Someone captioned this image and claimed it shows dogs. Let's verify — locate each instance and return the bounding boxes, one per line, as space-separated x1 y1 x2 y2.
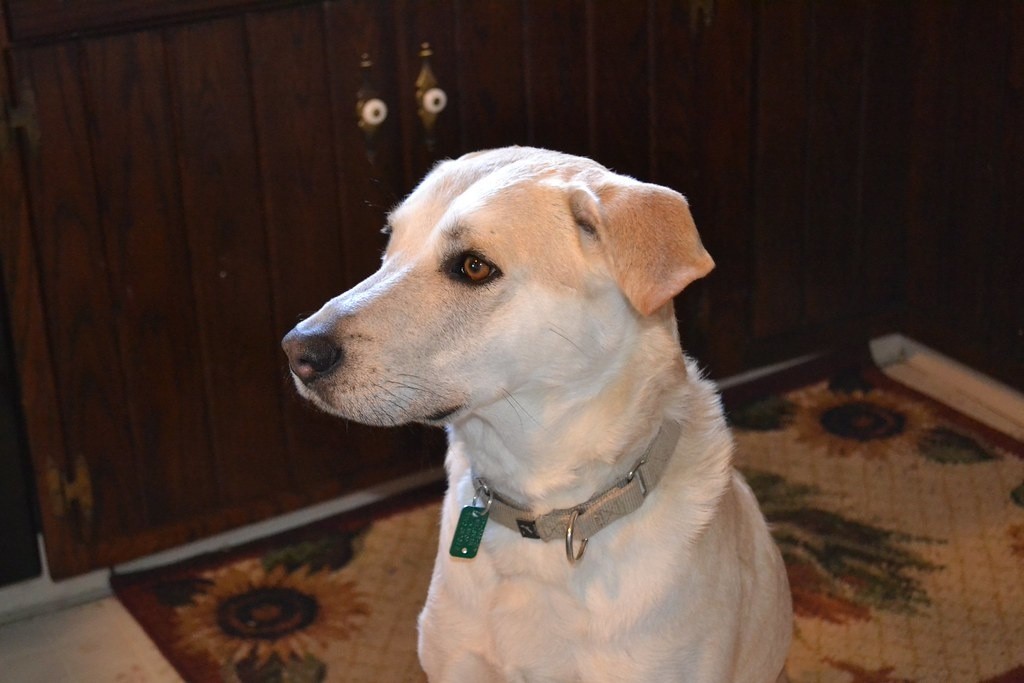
279 143 795 683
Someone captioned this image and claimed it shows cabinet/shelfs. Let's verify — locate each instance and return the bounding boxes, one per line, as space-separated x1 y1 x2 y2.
0 0 1024 567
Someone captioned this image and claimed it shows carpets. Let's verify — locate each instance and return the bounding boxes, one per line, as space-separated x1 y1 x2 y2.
110 330 1024 683
